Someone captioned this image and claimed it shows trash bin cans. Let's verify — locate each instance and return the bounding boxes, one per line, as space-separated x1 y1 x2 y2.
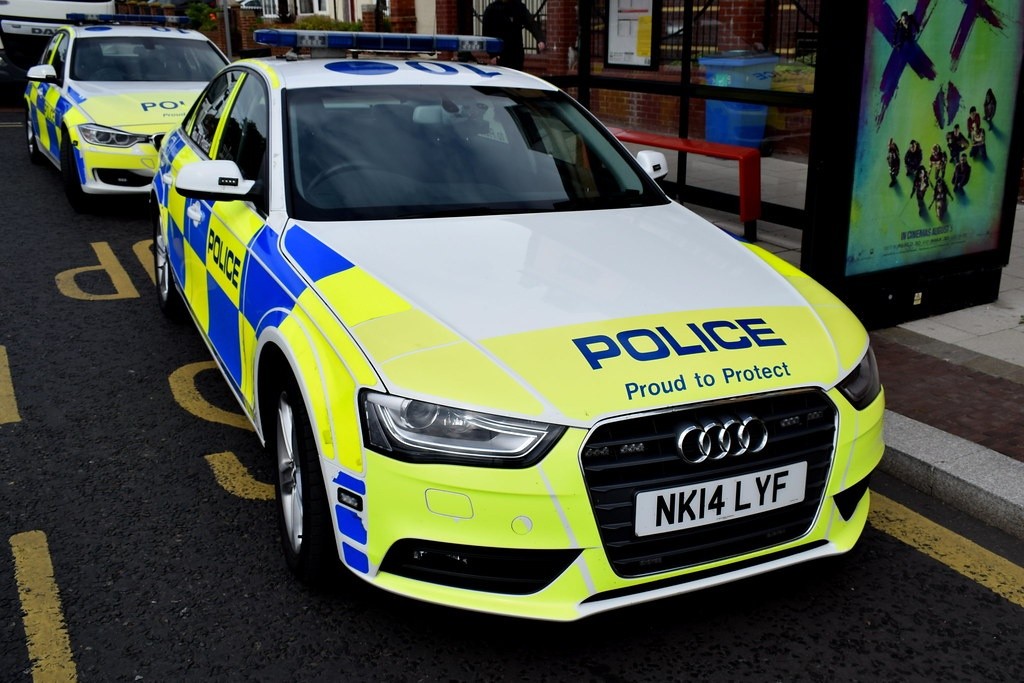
698 51 779 148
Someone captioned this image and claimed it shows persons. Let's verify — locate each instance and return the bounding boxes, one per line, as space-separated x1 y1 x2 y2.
910 165 935 222
970 120 988 162
897 10 911 29
905 140 922 176
968 106 980 138
139 42 192 81
297 103 439 195
983 89 996 130
77 42 105 78
928 144 947 179
887 139 901 187
929 178 953 220
946 124 969 165
483 0 546 71
951 153 971 197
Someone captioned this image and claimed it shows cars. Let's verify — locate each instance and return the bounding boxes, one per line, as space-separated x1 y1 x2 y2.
149 27 888 625
0 0 120 72
22 10 238 206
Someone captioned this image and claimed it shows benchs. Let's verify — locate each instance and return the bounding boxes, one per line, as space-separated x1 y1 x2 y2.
583 127 762 244
